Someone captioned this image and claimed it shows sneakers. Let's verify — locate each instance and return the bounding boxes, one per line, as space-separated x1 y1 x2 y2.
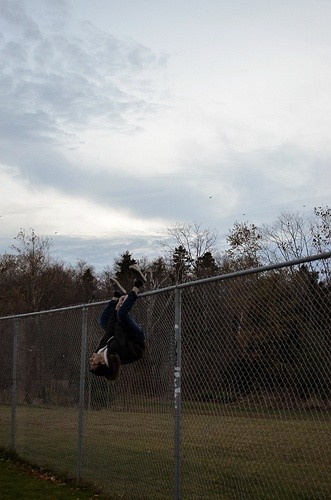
129 266 146 283
109 277 128 296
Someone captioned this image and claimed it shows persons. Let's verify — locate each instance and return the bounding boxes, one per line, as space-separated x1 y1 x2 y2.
85 263 149 381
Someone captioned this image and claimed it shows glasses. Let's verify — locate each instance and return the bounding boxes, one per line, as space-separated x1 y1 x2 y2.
89 362 99 366
